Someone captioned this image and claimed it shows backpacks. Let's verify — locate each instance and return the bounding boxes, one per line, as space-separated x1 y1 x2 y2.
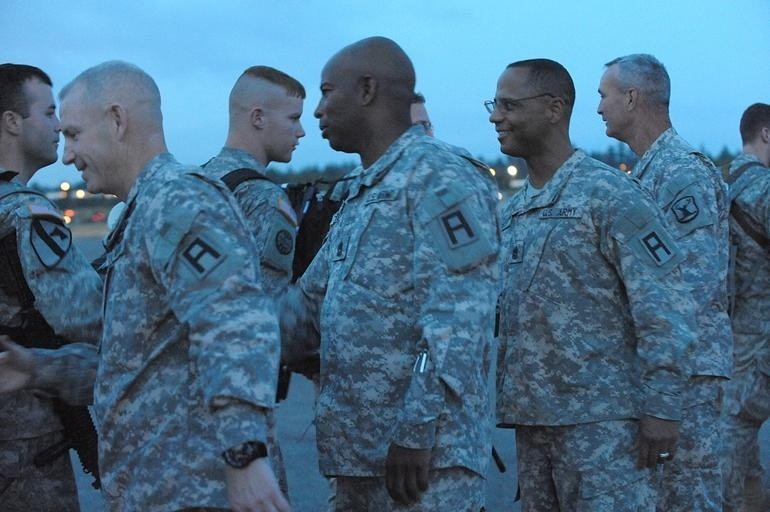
278 175 358 374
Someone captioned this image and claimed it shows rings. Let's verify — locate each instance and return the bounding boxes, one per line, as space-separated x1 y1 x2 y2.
659 449 671 458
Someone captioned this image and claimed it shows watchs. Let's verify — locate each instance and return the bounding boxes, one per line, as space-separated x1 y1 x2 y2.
223 440 266 470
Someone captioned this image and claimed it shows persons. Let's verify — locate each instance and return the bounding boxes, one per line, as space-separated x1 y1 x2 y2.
470 58 689 509
197 63 304 302
594 57 734 511
278 33 495 512
406 89 437 144
2 61 121 510
723 102 769 510
3 58 281 508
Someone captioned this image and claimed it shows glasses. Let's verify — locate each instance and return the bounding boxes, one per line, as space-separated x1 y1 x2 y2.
485 93 555 114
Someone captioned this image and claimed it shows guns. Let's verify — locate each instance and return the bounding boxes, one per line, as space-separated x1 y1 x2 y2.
3 228 102 489
